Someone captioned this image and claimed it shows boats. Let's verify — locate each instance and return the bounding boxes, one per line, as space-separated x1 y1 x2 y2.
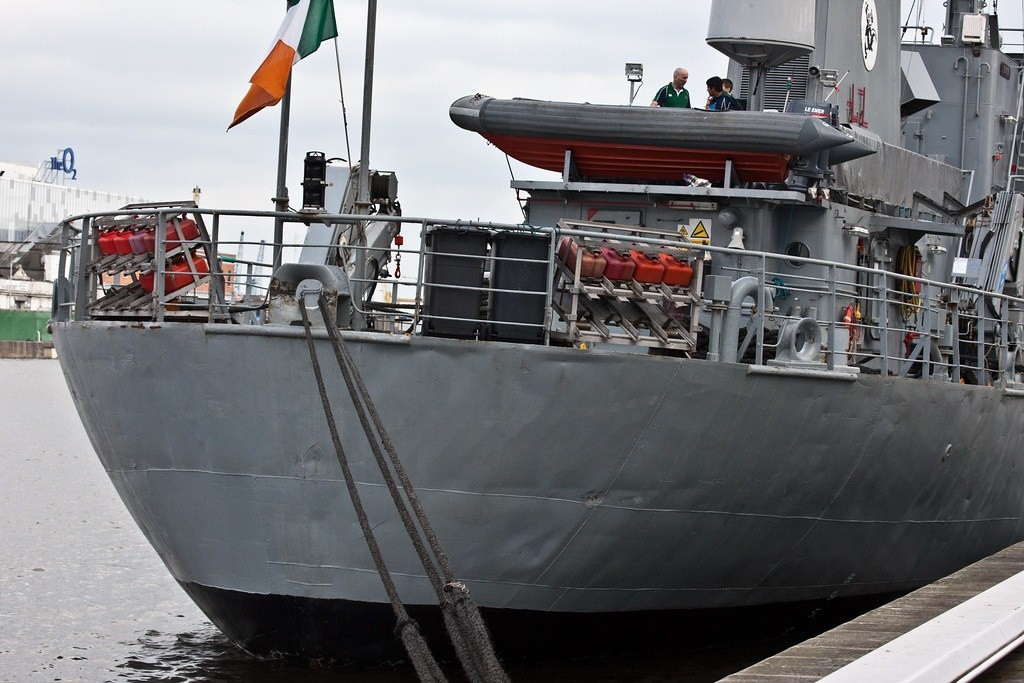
48 0 1024 674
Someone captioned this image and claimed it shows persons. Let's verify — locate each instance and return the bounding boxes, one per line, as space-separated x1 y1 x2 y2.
650 68 691 108
706 76 744 111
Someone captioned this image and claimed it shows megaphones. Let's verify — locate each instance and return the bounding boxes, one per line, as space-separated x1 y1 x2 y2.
808 66 820 77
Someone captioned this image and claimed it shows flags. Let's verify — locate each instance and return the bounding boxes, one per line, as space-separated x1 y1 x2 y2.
226 0 339 133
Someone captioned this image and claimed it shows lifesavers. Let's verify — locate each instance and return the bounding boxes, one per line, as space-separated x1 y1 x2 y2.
841 304 860 349
